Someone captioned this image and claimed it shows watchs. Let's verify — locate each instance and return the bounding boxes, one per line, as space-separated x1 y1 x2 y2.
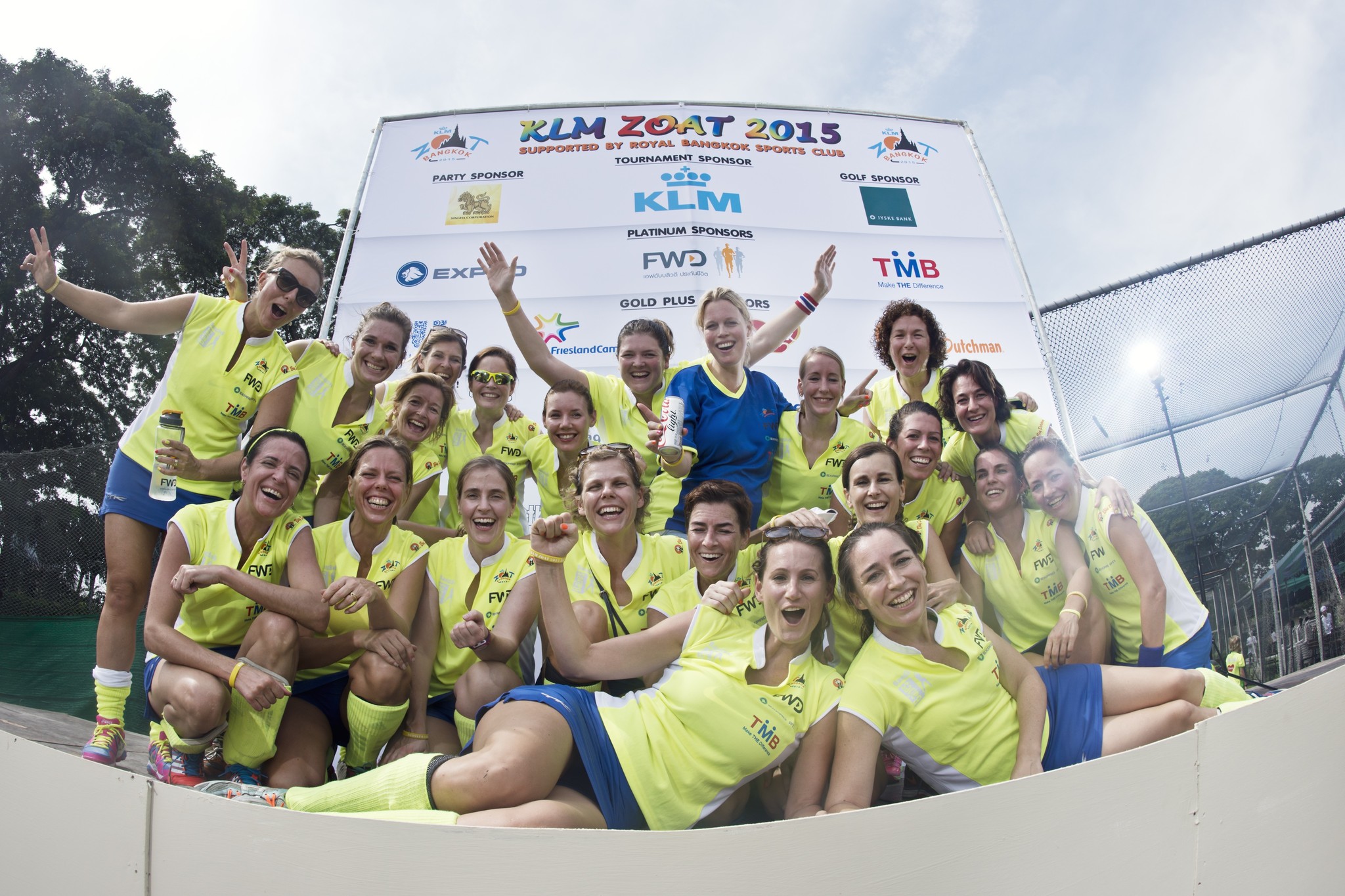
469 627 491 651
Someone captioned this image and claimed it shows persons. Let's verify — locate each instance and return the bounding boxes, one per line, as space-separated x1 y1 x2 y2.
861 299 1038 453
756 346 960 538
317 338 467 548
371 325 524 468
476 241 836 534
221 239 412 526
825 522 1286 817
142 427 332 786
383 456 542 764
524 380 648 532
960 444 1111 668
1226 605 1334 689
647 480 828 829
935 359 1135 556
437 346 543 537
749 442 972 677
1022 437 1212 669
20 226 324 784
193 510 840 829
827 401 970 565
266 436 430 788
635 286 878 541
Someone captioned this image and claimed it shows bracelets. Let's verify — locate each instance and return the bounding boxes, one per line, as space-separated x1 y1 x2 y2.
1139 644 1165 667
45 275 60 294
795 292 818 315
770 515 783 528
966 520 985 527
228 662 246 689
403 730 428 740
1065 591 1088 615
502 300 520 316
530 548 565 563
1059 609 1081 620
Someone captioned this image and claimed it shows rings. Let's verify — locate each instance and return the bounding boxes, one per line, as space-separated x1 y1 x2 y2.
639 455 642 460
174 577 177 580
350 592 358 602
1123 495 1128 499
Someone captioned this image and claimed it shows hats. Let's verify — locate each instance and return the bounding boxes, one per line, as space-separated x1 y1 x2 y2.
1320 606 1326 612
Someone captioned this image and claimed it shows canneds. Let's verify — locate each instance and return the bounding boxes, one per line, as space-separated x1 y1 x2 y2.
658 396 685 456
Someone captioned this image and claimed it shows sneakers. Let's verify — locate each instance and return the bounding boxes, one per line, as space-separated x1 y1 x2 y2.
146 731 289 809
336 759 376 780
1260 689 1287 697
82 715 127 767
881 748 903 780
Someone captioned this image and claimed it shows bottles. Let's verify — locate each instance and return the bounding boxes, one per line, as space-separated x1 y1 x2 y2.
149 409 186 502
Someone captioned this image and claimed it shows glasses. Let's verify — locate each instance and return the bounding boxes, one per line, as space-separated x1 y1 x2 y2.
424 325 467 348
471 369 514 385
266 267 318 309
761 526 829 552
577 442 636 465
